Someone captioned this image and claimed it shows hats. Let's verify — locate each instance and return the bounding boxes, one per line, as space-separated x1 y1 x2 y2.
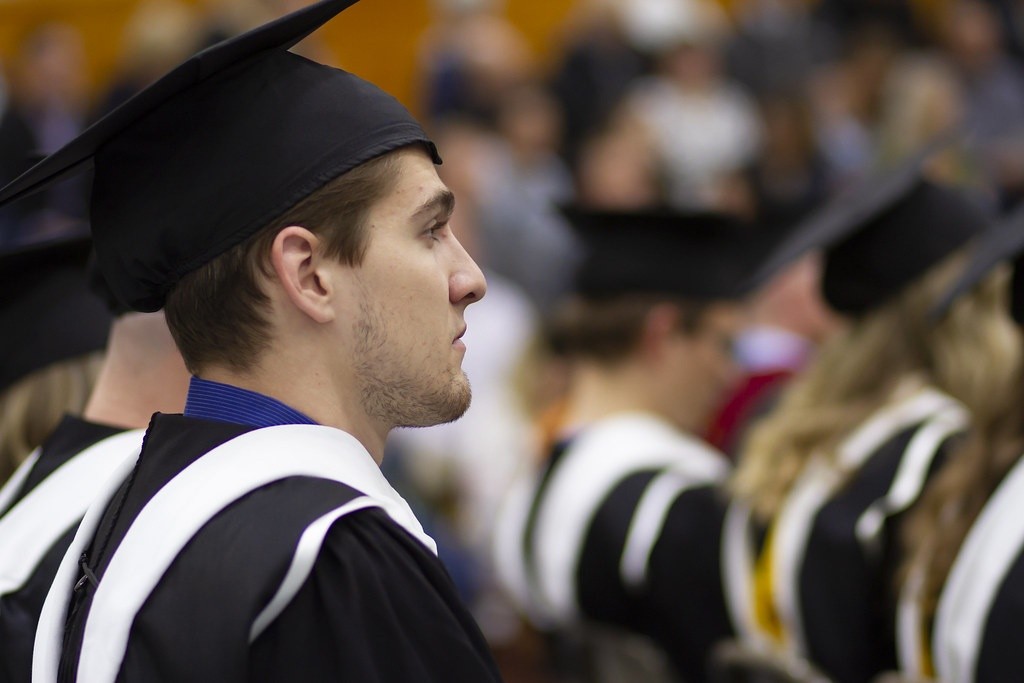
819 163 989 330
0 148 112 390
0 0 442 314
559 205 788 302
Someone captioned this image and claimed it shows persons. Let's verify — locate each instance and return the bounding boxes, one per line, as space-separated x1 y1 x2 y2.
30 0 502 682
0 0 1023 683
